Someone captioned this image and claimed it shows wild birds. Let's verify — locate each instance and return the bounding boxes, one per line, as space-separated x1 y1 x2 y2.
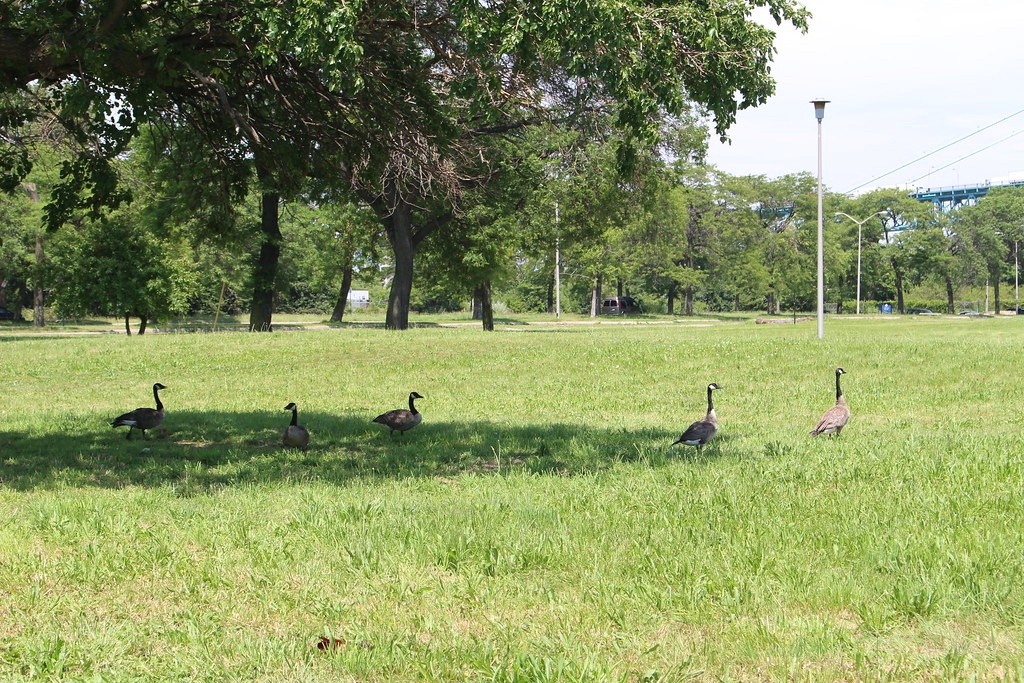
671 383 723 455
282 401 310 450
113 383 168 444
371 390 425 437
809 367 849 437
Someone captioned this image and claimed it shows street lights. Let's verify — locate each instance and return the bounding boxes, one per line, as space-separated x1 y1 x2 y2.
810 97 832 340
996 232 1022 316
834 209 887 315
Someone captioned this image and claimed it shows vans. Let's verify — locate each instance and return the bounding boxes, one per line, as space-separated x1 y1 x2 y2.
825 288 846 315
601 295 642 318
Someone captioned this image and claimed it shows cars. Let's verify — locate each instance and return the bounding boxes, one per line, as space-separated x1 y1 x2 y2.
1006 308 1024 314
906 308 932 315
958 310 979 315
